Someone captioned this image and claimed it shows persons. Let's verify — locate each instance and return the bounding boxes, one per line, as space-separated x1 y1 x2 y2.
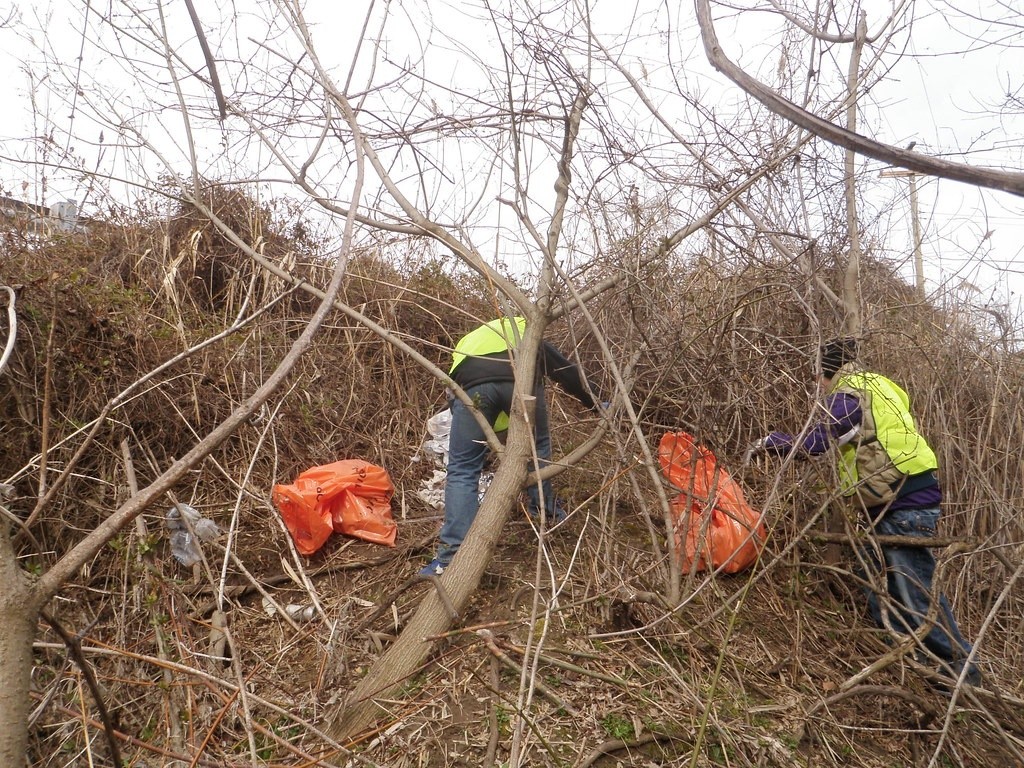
742 337 982 705
419 317 607 579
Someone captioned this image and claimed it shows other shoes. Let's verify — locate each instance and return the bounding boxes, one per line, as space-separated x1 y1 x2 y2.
923 662 982 698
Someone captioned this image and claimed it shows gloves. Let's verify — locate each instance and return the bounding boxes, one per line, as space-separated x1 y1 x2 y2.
742 435 768 468
594 403 609 418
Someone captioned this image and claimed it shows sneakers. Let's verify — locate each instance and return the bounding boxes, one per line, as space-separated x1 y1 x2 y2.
419 560 449 577
528 504 566 520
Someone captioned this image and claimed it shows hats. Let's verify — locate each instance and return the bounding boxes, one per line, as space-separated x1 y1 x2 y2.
819 337 859 377
